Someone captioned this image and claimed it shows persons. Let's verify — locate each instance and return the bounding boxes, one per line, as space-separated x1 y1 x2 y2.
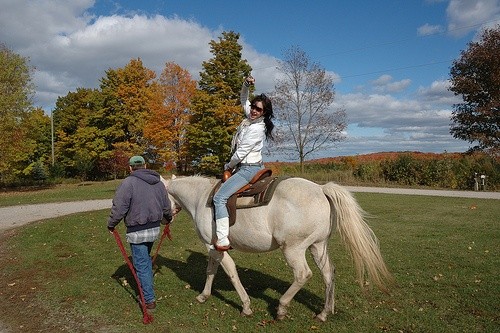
107 155 172 308
209 74 275 250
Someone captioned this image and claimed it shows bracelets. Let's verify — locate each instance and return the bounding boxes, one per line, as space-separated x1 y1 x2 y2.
243 82 249 87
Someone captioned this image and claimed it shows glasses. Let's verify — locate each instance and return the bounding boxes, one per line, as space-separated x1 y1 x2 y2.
251 103 263 112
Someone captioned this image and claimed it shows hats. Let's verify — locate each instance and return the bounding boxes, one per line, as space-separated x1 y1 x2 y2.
128 155 145 166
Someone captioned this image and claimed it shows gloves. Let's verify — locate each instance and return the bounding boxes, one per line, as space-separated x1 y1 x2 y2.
161 217 172 225
107 226 115 232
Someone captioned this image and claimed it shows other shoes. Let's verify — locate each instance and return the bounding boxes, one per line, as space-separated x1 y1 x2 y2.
145 302 156 309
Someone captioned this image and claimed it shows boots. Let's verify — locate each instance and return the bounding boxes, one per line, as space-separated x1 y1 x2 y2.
208 217 231 249
